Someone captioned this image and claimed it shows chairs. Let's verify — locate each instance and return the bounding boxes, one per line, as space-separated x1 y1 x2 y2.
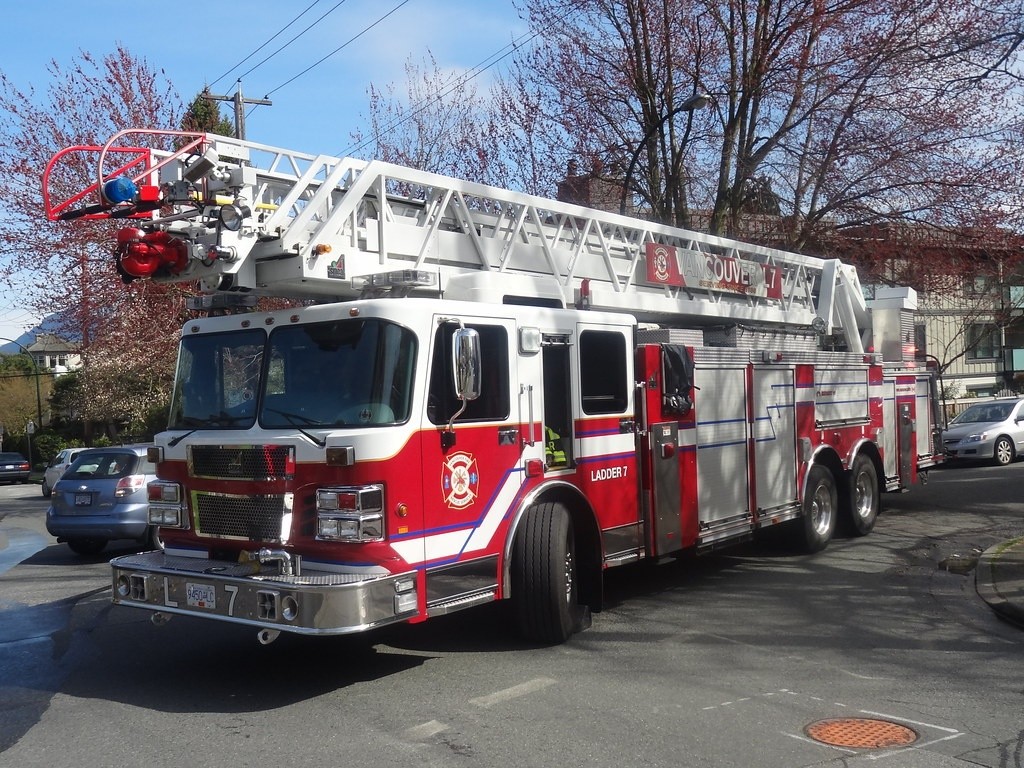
988 409 1003 421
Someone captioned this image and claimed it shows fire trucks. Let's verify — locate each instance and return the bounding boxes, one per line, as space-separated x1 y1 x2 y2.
41 129 938 646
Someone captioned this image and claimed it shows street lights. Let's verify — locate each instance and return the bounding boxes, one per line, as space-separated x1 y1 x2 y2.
616 91 710 214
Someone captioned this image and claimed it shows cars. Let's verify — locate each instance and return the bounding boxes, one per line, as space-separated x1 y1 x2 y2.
0 451 31 485
44 445 165 550
941 394 1024 465
41 448 97 495
